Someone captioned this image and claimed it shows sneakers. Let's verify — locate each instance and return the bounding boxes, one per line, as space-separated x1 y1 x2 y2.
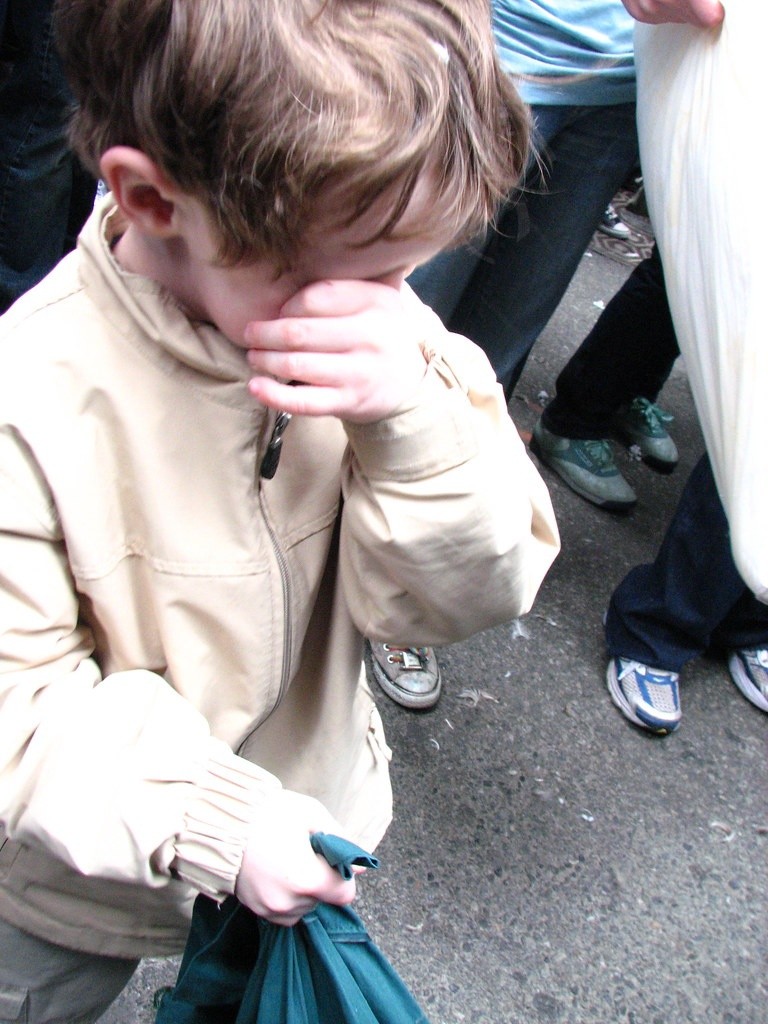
727 642 768 714
529 396 680 512
606 610 682 737
365 635 447 714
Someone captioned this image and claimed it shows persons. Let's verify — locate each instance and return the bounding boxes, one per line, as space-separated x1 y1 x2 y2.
0 0 104 315
530 242 683 509
1 1 564 1024
366 0 728 713
607 449 768 740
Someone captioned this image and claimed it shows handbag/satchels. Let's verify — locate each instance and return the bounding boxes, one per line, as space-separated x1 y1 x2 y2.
154 831 428 1024
